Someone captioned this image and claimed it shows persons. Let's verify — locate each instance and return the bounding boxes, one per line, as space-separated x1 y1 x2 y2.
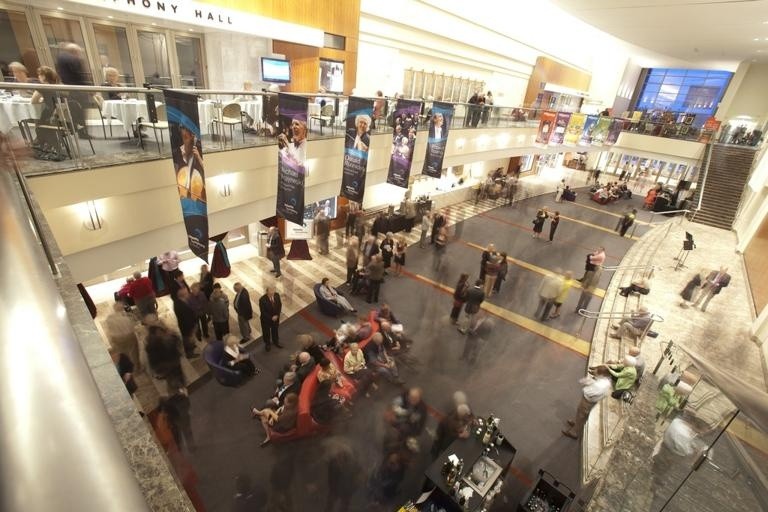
9 43 763 511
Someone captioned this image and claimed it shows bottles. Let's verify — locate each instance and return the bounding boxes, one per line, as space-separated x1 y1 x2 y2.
473 412 505 446
441 457 464 487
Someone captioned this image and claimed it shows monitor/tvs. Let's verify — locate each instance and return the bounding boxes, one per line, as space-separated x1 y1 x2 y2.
258 57 290 82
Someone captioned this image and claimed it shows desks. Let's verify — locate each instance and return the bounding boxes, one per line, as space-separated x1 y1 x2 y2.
425 423 517 512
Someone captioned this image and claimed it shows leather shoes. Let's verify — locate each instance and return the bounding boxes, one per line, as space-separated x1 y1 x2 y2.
351 309 357 312
562 418 577 438
191 353 200 357
276 343 283 348
239 338 248 344
270 270 281 278
204 335 209 338
266 345 271 351
198 338 201 341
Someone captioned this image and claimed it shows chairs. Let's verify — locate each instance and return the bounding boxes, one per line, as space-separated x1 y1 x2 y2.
373 99 388 130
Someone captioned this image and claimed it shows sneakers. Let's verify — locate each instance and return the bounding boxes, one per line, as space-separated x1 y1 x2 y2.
365 393 370 398
370 383 377 390
259 441 271 448
249 405 255 411
394 378 405 384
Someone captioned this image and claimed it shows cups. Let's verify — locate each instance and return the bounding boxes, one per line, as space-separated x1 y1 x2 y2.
5 92 12 100
0 92 7 101
12 90 20 100
